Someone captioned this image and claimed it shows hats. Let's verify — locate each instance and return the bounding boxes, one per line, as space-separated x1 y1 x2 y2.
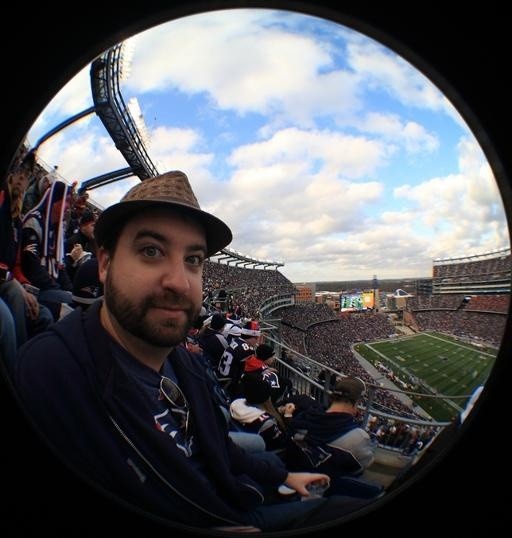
337 374 369 397
241 320 262 338
94 169 234 261
255 344 276 359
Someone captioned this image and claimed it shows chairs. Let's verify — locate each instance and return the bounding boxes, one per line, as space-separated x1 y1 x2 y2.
212 385 234 405
206 367 233 390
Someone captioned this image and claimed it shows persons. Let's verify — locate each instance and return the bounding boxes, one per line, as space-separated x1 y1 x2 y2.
0 141 512 534
